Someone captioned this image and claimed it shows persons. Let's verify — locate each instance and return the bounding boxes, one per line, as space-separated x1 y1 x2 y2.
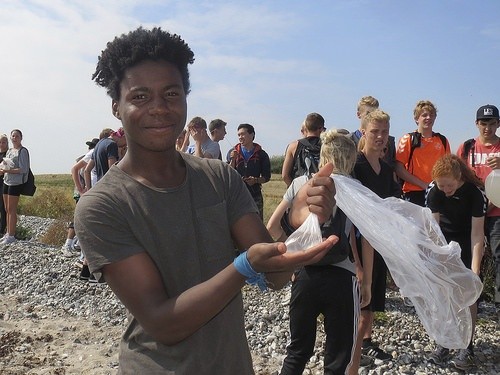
266 93 500 375
174 116 271 259
0 129 30 244
60 127 128 283
76 27 341 375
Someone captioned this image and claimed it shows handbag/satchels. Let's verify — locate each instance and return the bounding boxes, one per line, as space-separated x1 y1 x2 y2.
314 231 350 266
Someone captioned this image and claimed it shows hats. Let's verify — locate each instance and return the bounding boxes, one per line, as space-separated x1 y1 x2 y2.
86 139 99 146
476 104 499 119
209 119 226 131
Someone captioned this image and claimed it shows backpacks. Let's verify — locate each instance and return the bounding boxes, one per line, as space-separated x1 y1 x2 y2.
8 147 36 196
297 138 321 177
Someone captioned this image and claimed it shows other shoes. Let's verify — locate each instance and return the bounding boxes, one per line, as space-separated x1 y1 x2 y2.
404 297 414 307
88 274 107 284
80 269 90 279
0 235 7 242
0 237 15 244
61 245 74 257
70 246 81 256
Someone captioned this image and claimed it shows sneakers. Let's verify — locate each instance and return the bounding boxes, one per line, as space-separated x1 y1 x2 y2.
361 345 393 361
450 348 477 370
427 345 449 364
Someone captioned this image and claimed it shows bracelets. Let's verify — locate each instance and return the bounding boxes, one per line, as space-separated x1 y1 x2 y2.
280 207 295 237
234 252 270 292
